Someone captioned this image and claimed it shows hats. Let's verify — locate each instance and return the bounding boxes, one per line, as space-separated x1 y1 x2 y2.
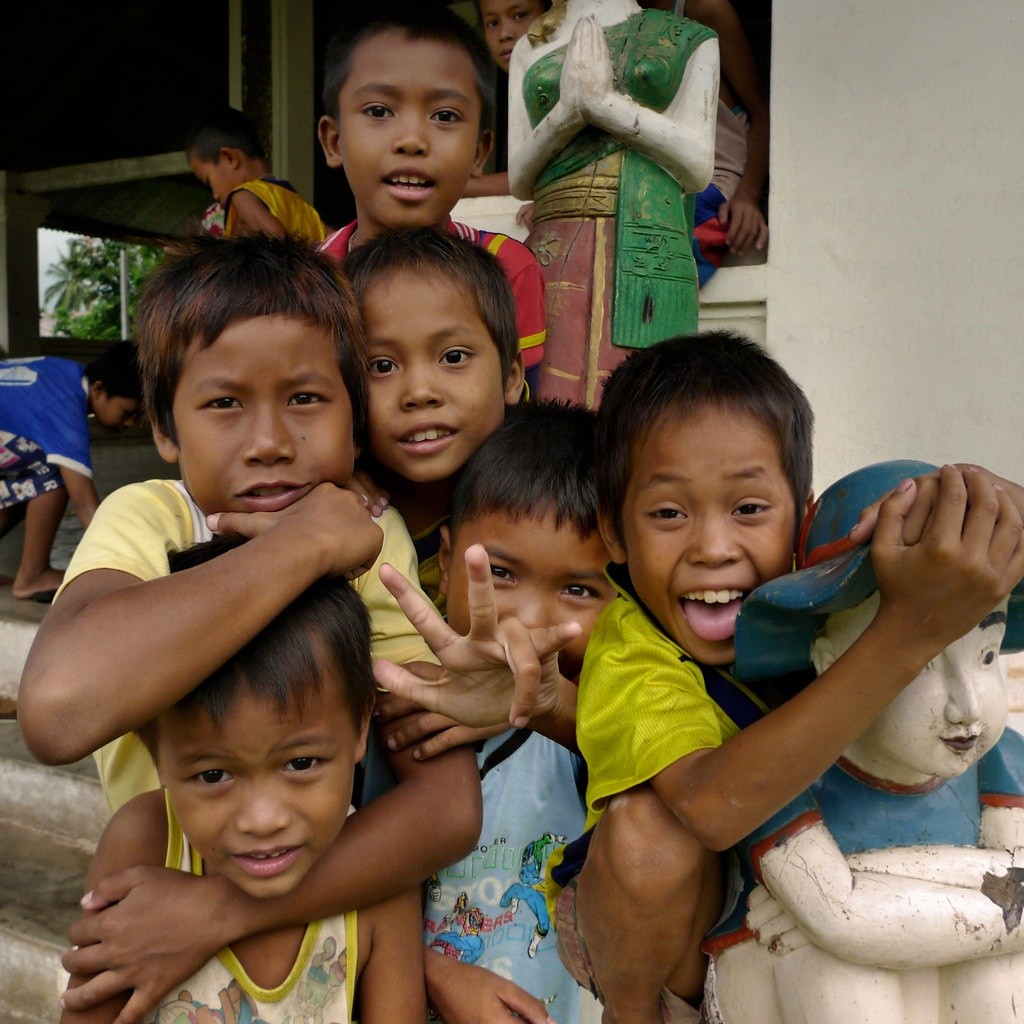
730 455 1024 681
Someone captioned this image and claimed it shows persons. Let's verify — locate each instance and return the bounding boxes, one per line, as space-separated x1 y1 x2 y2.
459 1 556 199
625 0 769 302
0 348 144 600
641 1 768 293
505 3 719 428
48 530 436 1024
568 330 1023 1024
315 2 548 406
186 103 336 267
16 228 484 1024
328 230 527 660
361 395 639 1024
697 459 1021 1024
